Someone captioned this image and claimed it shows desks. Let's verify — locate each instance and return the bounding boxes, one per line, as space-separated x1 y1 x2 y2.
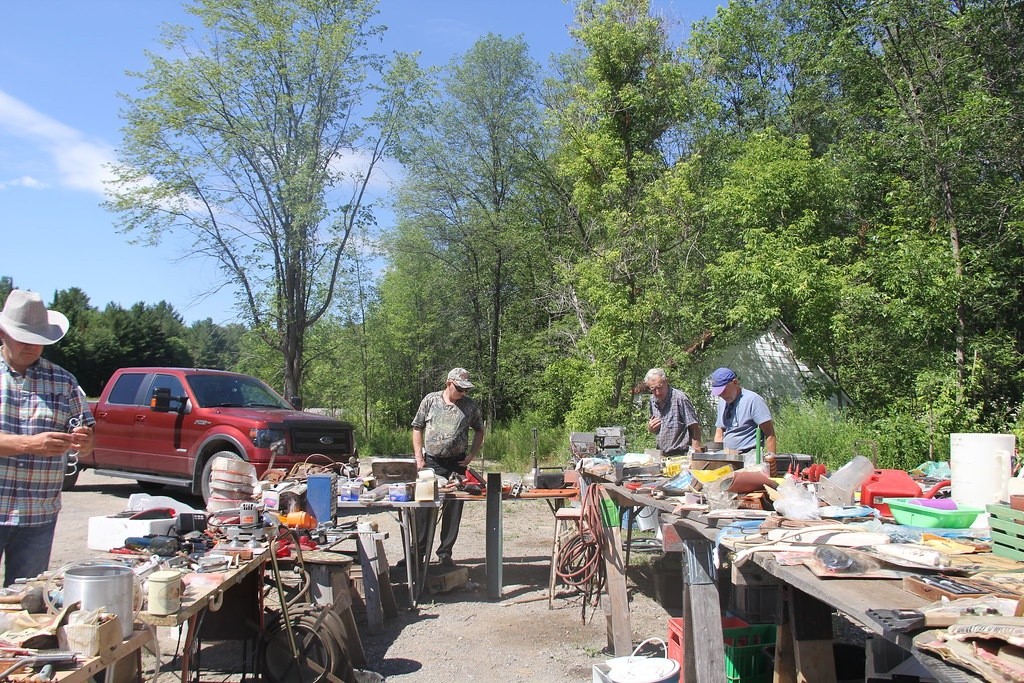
0 469 1024 683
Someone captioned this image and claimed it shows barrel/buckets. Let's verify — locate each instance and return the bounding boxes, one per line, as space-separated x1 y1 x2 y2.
608 638 680 683
691 453 744 492
63 566 134 638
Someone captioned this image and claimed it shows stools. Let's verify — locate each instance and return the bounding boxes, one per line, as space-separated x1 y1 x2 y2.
549 508 602 610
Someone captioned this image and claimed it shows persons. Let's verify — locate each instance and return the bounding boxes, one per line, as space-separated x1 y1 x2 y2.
711 368 776 454
397 368 483 568
1011 448 1024 477
0 289 97 588
644 368 701 457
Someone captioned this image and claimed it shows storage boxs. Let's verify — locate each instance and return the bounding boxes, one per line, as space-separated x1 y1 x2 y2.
667 618 777 683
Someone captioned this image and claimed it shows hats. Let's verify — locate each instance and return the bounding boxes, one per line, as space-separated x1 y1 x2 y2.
711 368 736 396
448 367 475 389
0 290 71 346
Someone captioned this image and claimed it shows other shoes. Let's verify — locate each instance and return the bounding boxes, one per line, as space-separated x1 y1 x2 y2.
397 555 422 565
439 555 455 568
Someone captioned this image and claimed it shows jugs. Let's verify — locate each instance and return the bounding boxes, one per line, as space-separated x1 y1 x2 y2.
950 433 1015 527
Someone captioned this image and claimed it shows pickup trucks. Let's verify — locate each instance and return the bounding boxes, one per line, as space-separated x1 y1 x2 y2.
62 367 360 507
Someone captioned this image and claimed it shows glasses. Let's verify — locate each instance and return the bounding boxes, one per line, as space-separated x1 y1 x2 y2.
451 382 465 392
726 402 734 419
